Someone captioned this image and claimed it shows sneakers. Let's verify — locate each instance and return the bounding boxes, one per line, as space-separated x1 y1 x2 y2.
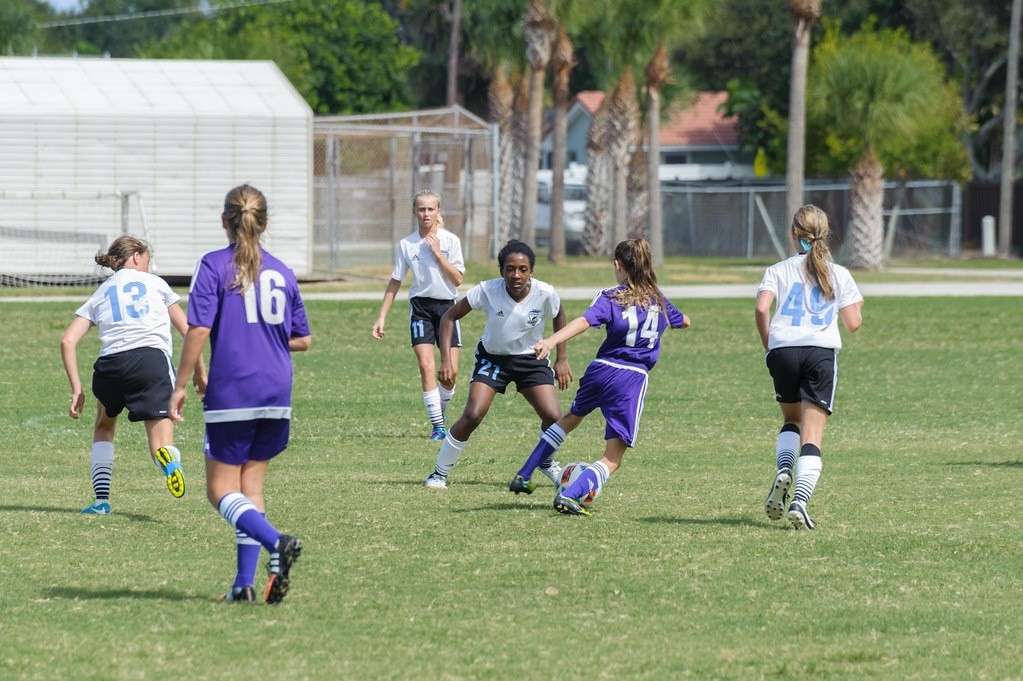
425 474 447 491
536 460 562 484
80 502 110 514
430 423 447 440
510 475 537 494
155 447 185 498
217 585 255 603
554 492 592 517
264 533 304 605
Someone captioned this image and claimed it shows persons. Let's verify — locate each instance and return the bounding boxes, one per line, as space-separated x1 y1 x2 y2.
509 239 691 516
373 192 466 441
427 242 573 489
170 185 310 603
62 237 208 514
755 205 865 531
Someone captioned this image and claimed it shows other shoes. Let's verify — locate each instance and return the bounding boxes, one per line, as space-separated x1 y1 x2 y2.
765 467 793 520
787 501 815 531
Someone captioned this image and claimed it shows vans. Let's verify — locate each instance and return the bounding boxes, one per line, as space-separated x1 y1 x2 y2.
531 142 755 256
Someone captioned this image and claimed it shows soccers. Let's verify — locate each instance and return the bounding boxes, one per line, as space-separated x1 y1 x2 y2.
555 461 601 507
